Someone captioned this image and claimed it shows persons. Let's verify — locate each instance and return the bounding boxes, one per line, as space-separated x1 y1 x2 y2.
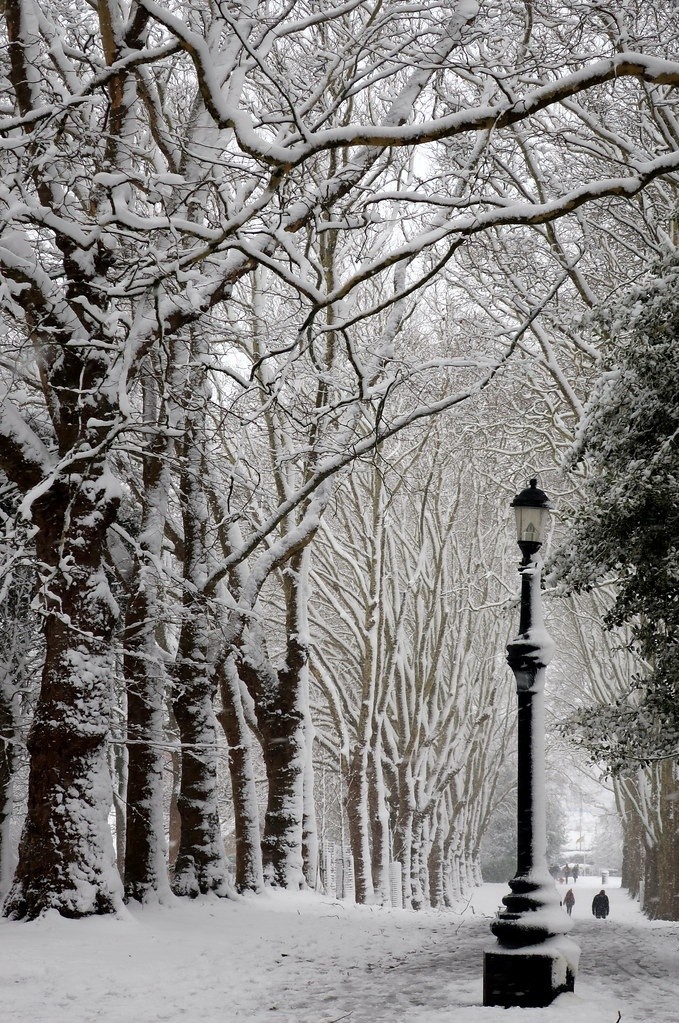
550 863 560 880
572 865 579 882
592 890 610 919
564 864 571 884
563 888 575 916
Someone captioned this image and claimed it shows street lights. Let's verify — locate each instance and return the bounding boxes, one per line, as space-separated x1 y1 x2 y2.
481 478 582 1011
